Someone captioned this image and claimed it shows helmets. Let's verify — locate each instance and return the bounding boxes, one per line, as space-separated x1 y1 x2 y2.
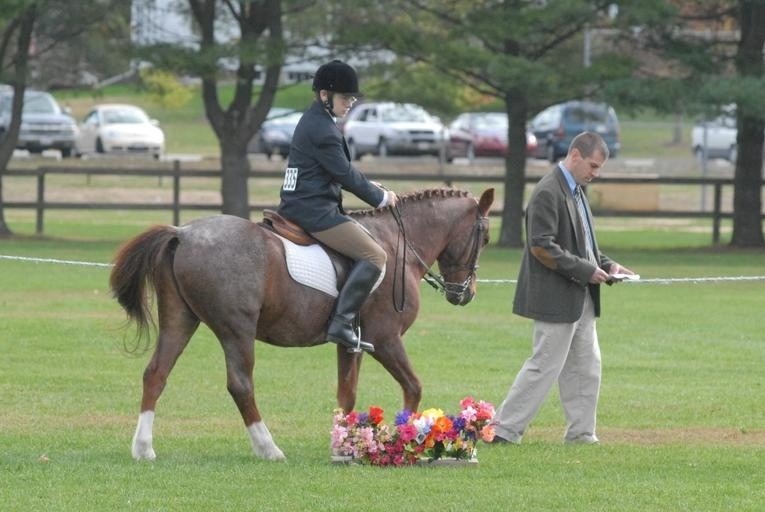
312 59 365 98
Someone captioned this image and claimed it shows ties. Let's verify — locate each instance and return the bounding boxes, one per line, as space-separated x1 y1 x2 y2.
575 185 599 267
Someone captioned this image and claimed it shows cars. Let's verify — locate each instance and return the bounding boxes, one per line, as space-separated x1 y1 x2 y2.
528 99 621 164
690 102 738 165
246 105 305 159
79 103 165 160
449 111 538 161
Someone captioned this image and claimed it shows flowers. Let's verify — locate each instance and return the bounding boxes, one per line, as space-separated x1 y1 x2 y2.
328 394 497 468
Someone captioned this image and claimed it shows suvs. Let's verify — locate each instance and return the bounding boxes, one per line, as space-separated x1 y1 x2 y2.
0 89 80 158
343 101 450 161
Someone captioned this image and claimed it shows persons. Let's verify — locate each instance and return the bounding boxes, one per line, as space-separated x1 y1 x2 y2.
277 60 399 353
487 131 635 444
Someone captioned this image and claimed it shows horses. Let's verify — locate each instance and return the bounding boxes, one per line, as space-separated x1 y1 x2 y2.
109 188 495 462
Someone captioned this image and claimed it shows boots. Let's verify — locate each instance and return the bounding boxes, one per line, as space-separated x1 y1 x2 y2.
325 258 382 353
